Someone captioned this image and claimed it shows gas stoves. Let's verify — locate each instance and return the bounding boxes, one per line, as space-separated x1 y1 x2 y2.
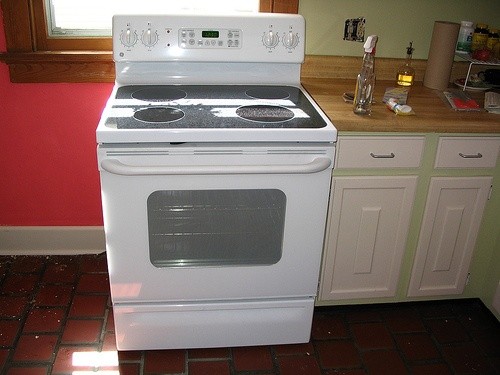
95 15 338 142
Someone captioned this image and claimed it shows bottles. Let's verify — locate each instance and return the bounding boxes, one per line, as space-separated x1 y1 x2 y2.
394 40 416 87
353 47 375 116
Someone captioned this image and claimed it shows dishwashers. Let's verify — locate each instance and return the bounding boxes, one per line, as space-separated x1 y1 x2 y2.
101 146 336 350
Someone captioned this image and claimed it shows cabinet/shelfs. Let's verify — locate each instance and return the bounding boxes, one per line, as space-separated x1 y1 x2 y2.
318 136 500 306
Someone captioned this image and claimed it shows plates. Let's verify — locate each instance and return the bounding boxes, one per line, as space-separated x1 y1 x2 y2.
455 78 491 94
